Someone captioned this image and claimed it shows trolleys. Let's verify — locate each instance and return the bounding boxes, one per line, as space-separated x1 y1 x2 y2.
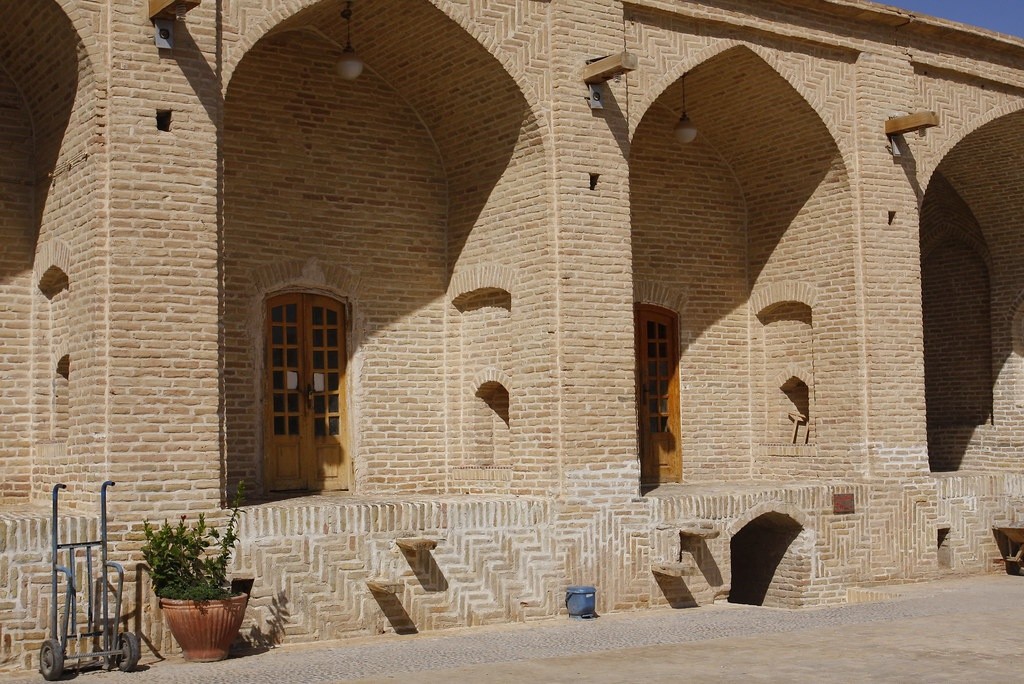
991 521 1024 577
37 480 141 681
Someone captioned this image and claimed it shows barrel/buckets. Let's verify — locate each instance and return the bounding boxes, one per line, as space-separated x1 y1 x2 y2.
565 585 597 621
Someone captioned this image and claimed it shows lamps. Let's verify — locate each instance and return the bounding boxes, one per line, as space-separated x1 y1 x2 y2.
673 73 697 144
335 0 363 80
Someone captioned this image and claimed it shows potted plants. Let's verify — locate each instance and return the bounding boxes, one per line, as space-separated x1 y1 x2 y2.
137 480 249 662
